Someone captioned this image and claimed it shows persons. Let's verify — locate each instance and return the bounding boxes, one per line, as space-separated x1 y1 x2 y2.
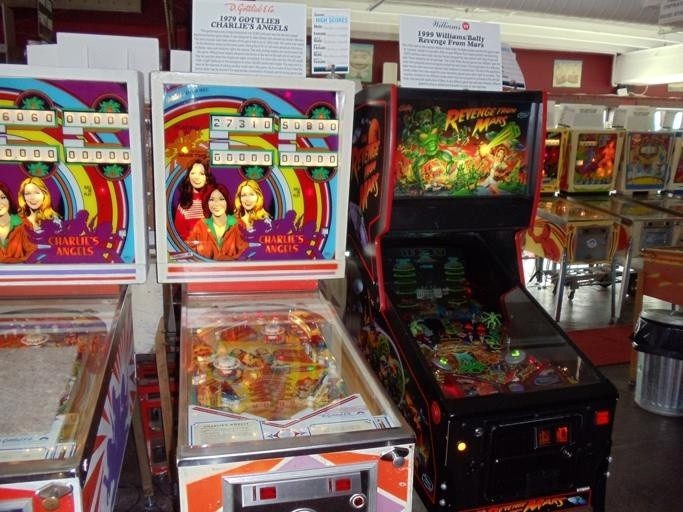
232 180 274 256
173 158 216 241
0 180 38 264
15 176 63 259
184 183 249 261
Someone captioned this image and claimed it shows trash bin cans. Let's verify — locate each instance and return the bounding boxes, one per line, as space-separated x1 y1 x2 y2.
631 309 683 417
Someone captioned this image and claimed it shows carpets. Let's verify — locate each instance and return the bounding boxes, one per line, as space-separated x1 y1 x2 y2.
567 325 635 368
